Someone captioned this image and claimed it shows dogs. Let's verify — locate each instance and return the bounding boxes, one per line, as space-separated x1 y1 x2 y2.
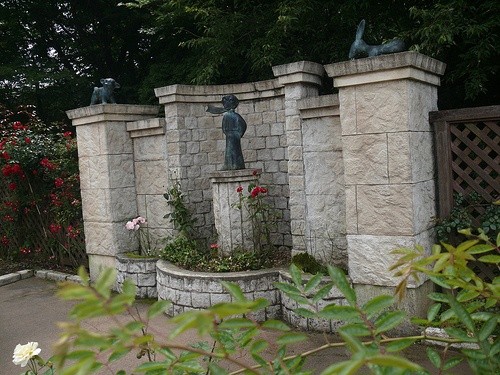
90 78 120 106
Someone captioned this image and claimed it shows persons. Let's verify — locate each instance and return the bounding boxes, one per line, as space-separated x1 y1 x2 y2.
220 95 248 168
